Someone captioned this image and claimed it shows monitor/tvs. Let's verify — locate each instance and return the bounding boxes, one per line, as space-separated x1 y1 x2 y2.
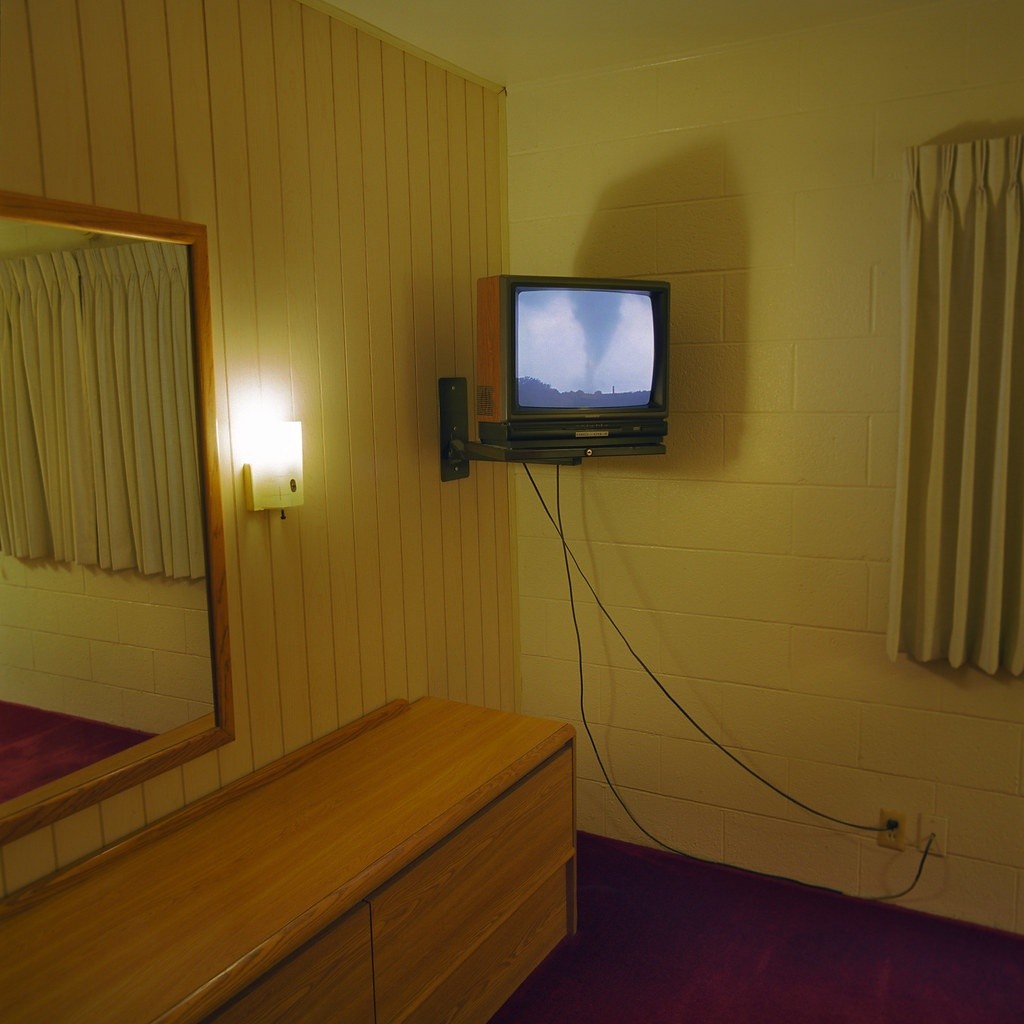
477 275 671 450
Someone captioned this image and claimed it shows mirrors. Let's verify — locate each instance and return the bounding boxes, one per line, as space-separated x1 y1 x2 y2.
0 188 237 848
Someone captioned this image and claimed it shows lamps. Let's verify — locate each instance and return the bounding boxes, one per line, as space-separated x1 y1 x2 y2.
244 421 304 511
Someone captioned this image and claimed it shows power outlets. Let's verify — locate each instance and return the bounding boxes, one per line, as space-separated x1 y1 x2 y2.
877 808 906 852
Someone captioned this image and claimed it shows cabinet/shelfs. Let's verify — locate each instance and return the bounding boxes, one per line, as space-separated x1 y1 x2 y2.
0 699 579 1024
